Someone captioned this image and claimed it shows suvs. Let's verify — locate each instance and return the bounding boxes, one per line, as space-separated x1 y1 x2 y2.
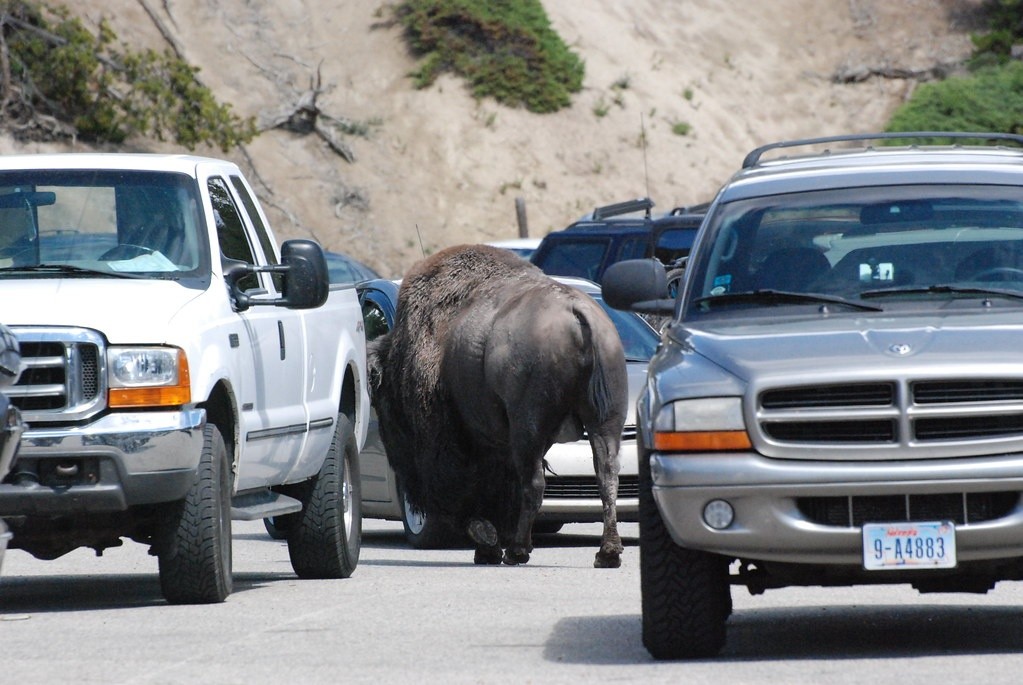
602 130 1023 665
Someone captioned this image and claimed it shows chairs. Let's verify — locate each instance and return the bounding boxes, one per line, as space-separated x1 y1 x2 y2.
120 208 193 268
765 247 832 293
955 248 1023 282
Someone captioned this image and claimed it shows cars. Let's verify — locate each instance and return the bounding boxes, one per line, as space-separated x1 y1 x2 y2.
320 199 1023 551
1 229 117 255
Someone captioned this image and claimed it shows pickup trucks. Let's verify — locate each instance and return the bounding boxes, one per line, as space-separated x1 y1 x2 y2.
0 154 373 605
